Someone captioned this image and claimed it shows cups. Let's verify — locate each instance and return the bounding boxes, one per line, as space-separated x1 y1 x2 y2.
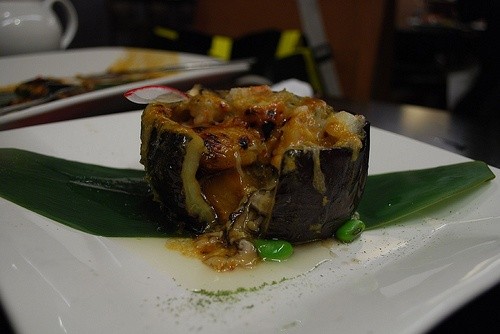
0 0 77 53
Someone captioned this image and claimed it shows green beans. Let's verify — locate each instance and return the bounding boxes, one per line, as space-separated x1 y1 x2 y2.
336 219 365 241
251 239 293 259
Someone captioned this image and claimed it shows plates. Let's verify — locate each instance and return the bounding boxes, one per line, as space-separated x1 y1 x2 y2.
0 109 500 334
0 48 250 125
